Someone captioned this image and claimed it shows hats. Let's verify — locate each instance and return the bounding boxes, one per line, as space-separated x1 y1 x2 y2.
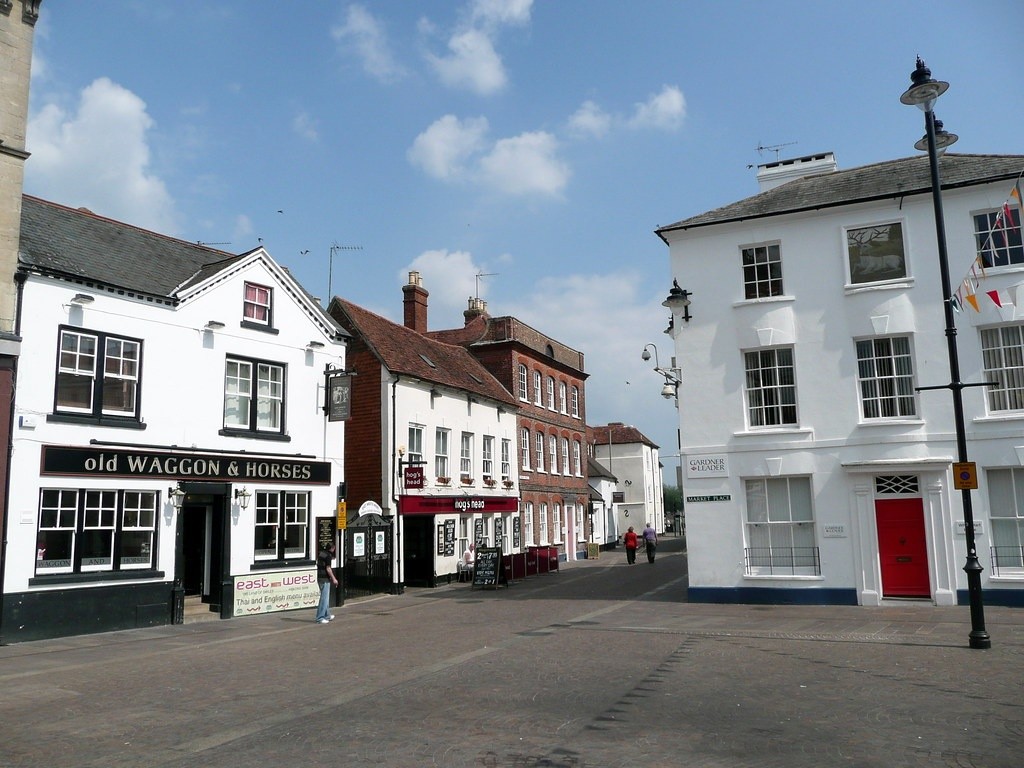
628 526 634 530
470 544 474 550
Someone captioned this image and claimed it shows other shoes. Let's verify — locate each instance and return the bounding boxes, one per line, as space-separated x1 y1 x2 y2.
317 619 329 623
629 563 631 564
632 561 635 564
649 561 654 564
327 615 335 620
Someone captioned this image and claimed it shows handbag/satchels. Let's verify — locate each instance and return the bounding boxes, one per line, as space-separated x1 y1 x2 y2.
623 533 627 545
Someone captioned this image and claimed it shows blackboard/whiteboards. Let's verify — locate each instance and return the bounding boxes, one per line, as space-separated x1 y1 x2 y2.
472 547 506 586
588 543 599 558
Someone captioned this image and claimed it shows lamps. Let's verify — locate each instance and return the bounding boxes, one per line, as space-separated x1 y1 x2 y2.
430 389 442 398
70 294 95 305
497 407 507 415
168 483 188 510
204 320 225 330
423 476 428 486
469 397 480 404
235 486 252 511
661 277 693 323
663 313 674 340
654 368 682 399
642 343 658 369
625 480 632 487
306 341 325 349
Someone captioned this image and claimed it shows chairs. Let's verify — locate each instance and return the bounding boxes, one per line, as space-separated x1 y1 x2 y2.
457 561 471 583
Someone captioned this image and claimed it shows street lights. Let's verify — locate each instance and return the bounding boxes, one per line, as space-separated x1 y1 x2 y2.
902 51 993 650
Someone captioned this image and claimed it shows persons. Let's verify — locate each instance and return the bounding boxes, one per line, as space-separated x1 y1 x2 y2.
462 544 477 585
315 543 338 624
623 527 638 564
643 523 658 564
